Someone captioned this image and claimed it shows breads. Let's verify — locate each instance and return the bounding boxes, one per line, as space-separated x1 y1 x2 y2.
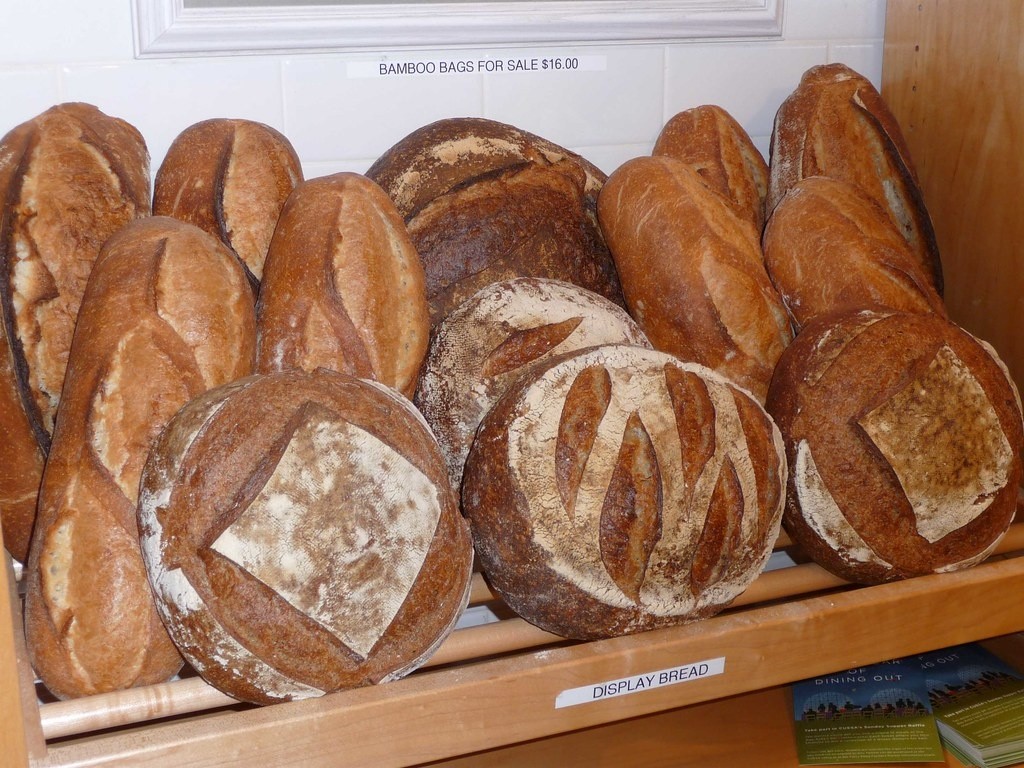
0 62 1024 702
